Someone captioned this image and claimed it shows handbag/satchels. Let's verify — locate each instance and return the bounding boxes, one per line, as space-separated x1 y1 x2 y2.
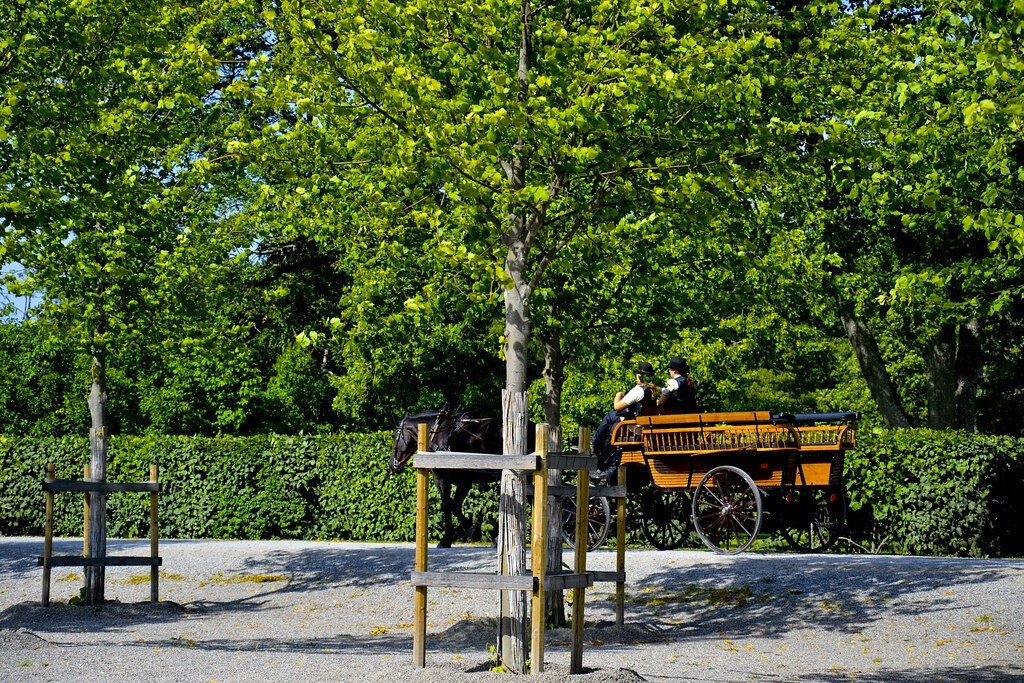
677 379 697 410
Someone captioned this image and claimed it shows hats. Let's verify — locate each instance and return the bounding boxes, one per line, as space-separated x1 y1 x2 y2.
634 363 655 374
666 357 690 370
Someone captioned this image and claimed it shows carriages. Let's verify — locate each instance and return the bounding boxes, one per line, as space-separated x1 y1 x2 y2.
390 404 866 556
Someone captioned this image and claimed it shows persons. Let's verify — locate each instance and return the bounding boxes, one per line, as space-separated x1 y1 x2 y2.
613 361 656 452
656 356 697 446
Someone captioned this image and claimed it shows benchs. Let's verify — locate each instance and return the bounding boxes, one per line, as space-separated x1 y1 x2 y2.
642 425 849 454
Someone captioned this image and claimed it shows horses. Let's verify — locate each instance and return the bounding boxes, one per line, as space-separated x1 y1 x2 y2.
390 410 537 549
